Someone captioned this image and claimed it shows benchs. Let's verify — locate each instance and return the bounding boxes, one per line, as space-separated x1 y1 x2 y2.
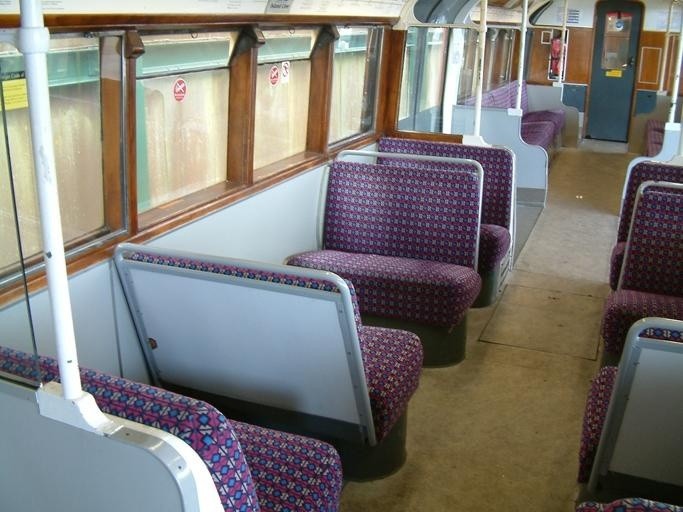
1 349 343 511
579 116 682 506
464 78 564 167
114 243 423 447
285 150 483 360
377 138 515 306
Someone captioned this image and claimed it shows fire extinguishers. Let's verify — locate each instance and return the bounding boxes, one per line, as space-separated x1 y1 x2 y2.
550 37 564 77
